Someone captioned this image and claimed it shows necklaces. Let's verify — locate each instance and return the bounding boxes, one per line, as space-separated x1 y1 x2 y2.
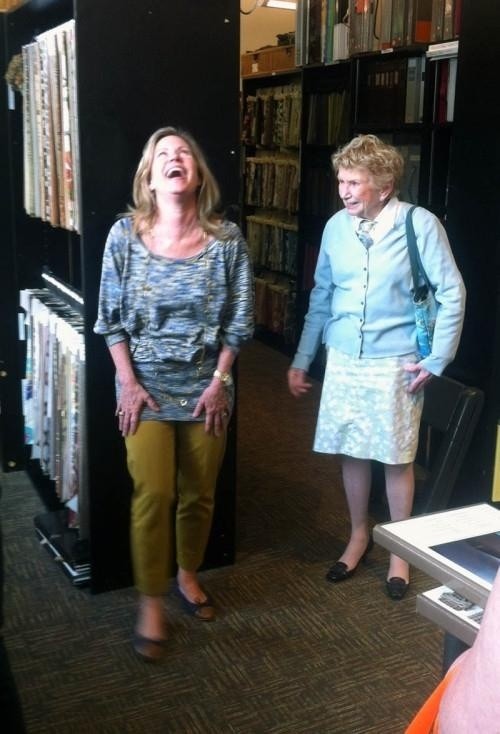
147 247 212 407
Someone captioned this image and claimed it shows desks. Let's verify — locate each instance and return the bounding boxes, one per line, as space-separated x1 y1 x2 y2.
370 501 500 680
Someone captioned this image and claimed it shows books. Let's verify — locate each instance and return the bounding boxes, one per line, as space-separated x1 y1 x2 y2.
240 0 462 361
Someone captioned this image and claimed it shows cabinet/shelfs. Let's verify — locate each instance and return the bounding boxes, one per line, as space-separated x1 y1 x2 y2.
1 0 235 593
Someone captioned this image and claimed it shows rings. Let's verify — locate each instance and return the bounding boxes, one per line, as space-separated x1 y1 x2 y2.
119 408 126 419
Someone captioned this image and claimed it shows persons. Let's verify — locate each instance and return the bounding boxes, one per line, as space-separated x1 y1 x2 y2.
436 550 499 731
93 124 260 662
288 130 470 593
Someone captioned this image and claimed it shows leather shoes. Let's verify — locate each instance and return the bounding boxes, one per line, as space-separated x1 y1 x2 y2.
175 575 219 622
129 591 172 663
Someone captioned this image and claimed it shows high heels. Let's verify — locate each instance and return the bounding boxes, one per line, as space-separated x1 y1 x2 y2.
326 526 375 584
385 574 410 602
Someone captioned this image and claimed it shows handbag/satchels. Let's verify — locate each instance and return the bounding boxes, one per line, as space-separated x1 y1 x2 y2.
408 282 440 361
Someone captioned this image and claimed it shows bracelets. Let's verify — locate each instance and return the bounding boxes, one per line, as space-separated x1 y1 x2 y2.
213 367 231 384
